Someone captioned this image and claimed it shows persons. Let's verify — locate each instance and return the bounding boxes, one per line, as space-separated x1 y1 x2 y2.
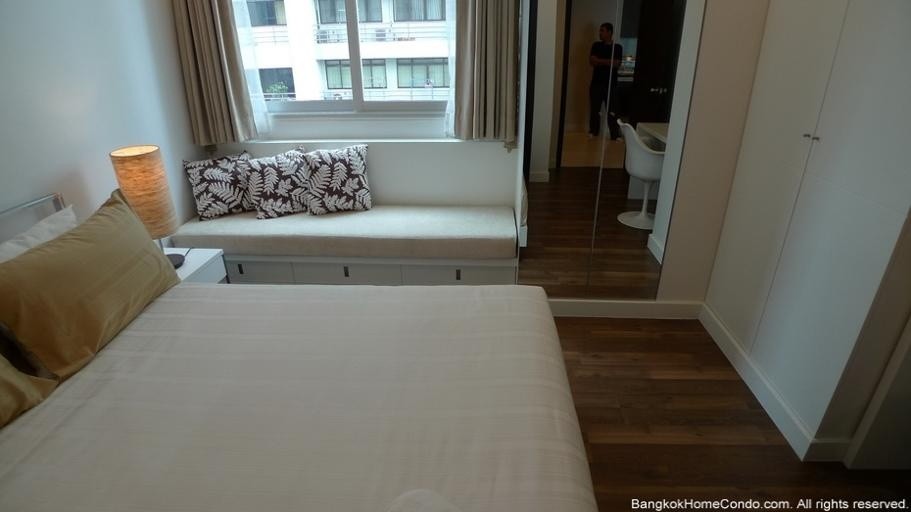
587 21 624 143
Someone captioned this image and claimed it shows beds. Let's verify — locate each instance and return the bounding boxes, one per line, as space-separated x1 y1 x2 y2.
0 192 598 511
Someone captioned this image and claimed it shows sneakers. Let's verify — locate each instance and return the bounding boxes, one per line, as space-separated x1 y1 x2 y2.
616 136 623 141
586 133 594 139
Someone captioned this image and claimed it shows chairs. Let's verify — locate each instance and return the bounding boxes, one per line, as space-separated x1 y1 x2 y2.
612 118 666 232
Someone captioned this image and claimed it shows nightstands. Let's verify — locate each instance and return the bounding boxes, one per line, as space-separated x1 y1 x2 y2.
158 247 228 285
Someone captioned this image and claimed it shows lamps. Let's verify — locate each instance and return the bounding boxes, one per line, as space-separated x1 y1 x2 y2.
111 142 184 269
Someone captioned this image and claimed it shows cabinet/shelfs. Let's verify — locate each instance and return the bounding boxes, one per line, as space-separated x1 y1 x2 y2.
699 0 910 458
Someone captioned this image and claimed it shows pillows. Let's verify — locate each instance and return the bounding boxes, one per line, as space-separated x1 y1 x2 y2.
182 144 371 218
0 186 181 439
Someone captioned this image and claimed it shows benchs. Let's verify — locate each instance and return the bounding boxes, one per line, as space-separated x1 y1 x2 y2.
173 199 518 286
